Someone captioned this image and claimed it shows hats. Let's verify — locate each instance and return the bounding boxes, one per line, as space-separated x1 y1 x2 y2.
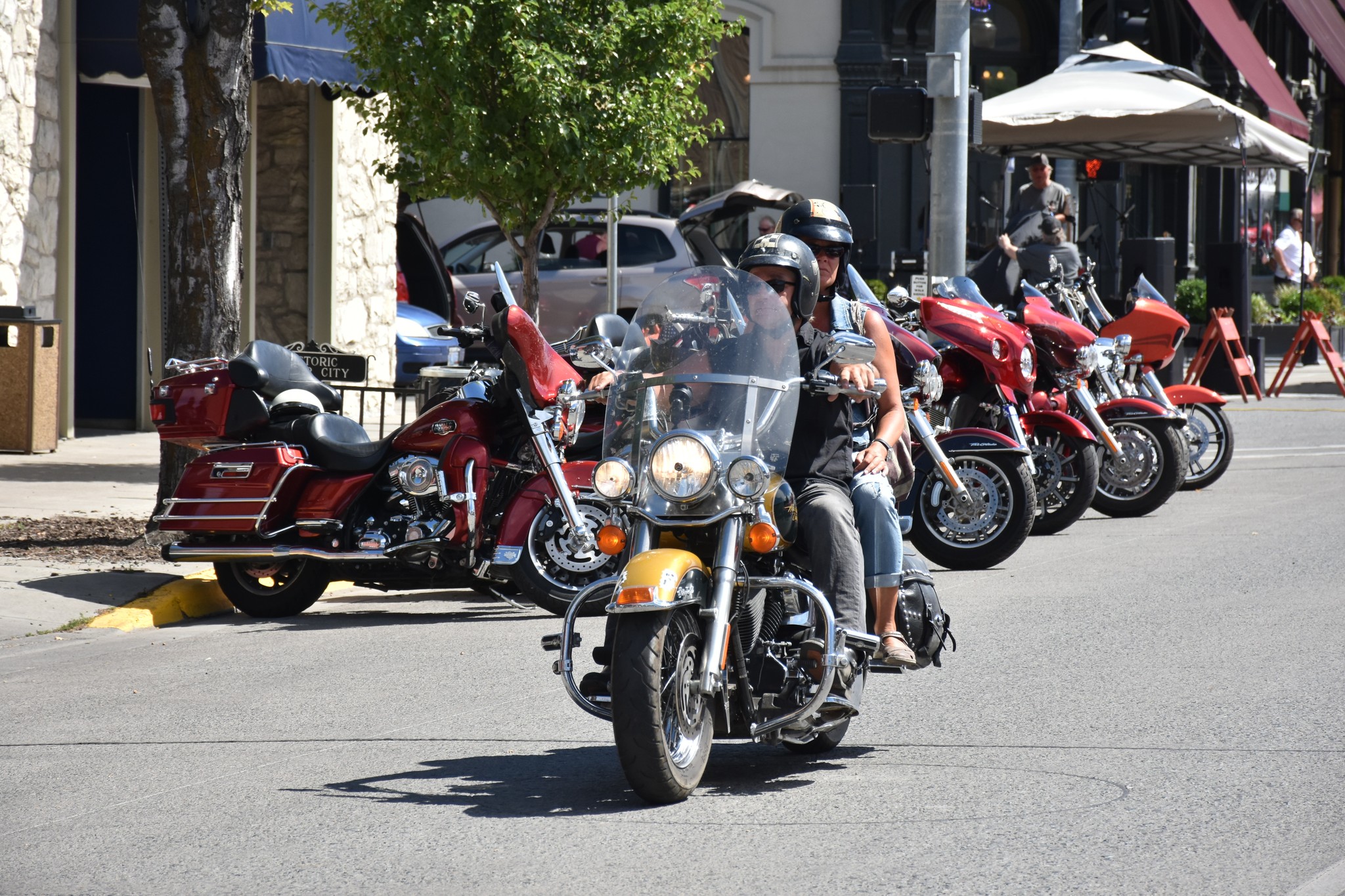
1038 216 1063 235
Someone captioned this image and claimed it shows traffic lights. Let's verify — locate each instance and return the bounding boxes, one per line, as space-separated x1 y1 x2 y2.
1074 159 1126 185
866 86 929 143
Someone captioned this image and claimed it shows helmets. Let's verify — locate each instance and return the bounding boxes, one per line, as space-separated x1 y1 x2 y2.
774 199 854 287
734 233 820 317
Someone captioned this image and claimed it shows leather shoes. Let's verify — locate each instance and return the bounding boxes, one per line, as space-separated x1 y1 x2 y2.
803 637 863 690
580 666 611 697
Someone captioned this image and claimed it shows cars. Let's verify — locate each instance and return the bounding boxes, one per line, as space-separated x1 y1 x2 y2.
398 172 465 325
397 302 464 387
440 178 809 370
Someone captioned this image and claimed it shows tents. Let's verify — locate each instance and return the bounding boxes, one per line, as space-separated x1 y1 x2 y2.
927 40 1331 358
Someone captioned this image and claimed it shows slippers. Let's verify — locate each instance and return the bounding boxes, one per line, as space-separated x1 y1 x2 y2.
876 630 918 664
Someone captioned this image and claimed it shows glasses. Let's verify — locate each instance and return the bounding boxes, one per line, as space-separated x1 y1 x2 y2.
758 227 769 232
807 242 845 258
747 279 795 295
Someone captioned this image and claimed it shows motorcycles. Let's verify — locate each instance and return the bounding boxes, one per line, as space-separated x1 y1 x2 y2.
144 253 1238 618
539 264 909 804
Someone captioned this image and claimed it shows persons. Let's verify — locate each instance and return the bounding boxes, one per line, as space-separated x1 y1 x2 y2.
773 198 916 665
1262 212 1273 248
1005 153 1075 235
1274 208 1303 288
1298 228 1317 289
758 216 775 236
579 232 881 697
998 216 1082 312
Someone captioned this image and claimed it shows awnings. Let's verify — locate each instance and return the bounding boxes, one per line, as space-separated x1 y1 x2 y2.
76 0 429 101
1186 0 1309 140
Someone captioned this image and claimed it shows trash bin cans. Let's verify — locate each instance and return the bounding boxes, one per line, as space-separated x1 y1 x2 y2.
417 365 486 415
0 304 63 455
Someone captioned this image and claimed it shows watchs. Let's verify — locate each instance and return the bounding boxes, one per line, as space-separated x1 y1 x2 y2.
869 439 893 462
